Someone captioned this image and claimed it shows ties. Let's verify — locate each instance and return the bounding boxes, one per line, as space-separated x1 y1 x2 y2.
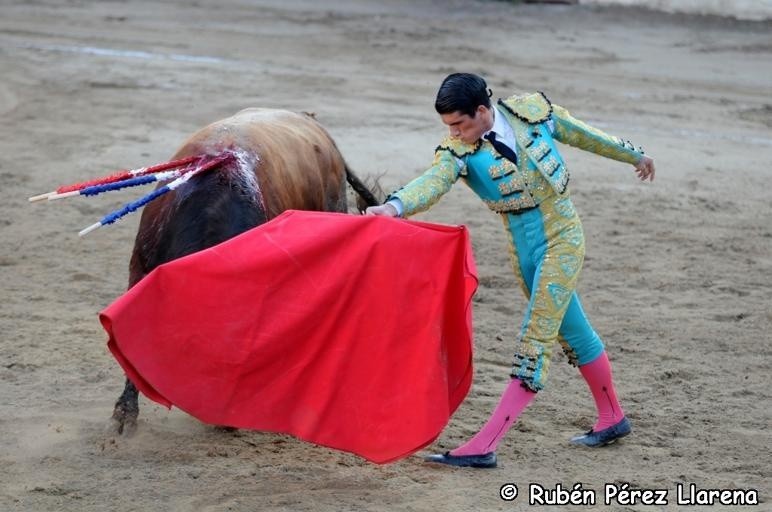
484 132 517 164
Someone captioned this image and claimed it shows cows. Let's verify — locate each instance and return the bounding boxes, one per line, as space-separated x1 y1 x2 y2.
106 103 382 438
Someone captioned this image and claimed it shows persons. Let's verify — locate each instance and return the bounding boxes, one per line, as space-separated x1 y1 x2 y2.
361 73 655 469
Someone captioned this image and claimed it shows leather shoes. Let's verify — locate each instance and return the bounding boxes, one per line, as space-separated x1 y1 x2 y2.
424 451 497 468
571 416 631 447
